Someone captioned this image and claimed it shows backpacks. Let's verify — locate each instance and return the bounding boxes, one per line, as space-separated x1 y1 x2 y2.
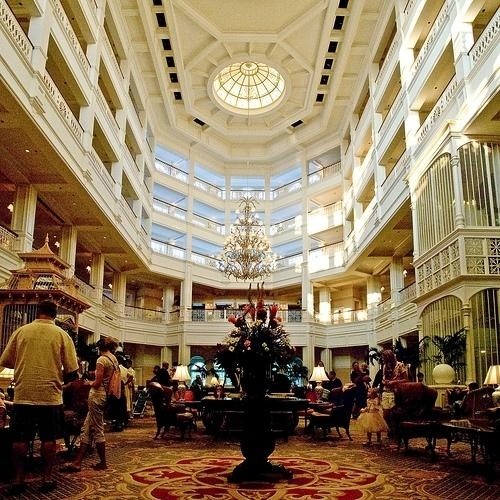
101 355 126 409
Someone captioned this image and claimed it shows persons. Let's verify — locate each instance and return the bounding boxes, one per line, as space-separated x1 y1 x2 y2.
460 383 479 408
359 362 370 389
303 383 352 434
356 391 392 447
0 301 80 496
444 386 468 415
58 339 121 474
308 360 330 390
151 366 161 382
323 371 342 390
208 384 233 401
125 359 137 421
381 349 410 437
417 372 424 382
351 361 370 421
111 355 132 433
191 374 204 401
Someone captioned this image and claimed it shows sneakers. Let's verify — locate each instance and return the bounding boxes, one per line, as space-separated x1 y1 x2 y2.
65 462 81 473
42 480 57 492
91 462 107 469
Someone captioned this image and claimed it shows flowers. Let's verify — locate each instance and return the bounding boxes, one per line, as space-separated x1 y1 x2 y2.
216 281 297 377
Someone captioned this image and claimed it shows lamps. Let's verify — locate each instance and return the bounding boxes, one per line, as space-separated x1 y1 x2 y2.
215 188 286 284
307 364 331 403
483 365 500 407
171 362 192 402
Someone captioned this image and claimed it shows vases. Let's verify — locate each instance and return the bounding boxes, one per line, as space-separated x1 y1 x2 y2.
239 364 271 397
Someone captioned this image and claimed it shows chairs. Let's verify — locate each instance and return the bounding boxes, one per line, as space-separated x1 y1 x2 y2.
147 381 359 443
382 382 494 454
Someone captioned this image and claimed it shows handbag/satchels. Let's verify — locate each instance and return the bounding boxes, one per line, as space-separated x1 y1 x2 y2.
381 391 396 409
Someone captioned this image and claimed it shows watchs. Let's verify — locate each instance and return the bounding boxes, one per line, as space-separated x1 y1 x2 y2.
157 361 173 388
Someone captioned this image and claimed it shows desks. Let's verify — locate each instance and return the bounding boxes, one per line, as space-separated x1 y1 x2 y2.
200 394 309 484
